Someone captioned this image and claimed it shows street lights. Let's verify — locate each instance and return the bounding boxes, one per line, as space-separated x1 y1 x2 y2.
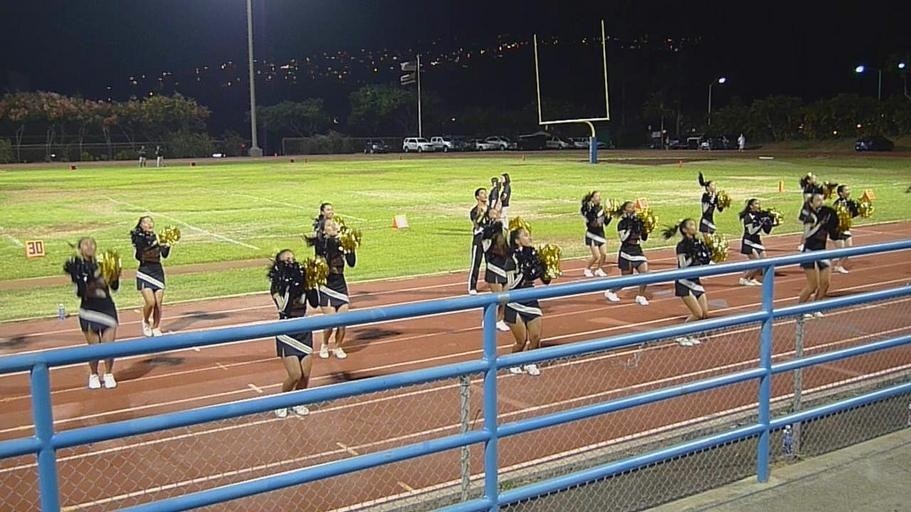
855 62 906 101
708 77 727 127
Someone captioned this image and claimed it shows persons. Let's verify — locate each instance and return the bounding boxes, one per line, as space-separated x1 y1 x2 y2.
738 199 777 285
695 171 724 266
604 201 649 305
738 132 746 152
468 173 561 376
581 191 612 278
661 218 712 345
312 216 356 359
792 170 876 319
133 215 170 338
155 144 165 168
269 249 320 419
138 145 147 168
315 203 334 230
65 236 118 389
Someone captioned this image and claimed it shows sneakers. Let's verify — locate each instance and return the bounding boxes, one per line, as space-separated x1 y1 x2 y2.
739 278 762 286
595 268 607 277
509 366 523 374
292 405 309 416
676 336 700 346
468 289 477 294
605 290 620 301
332 344 347 359
494 319 510 331
143 320 162 337
89 373 101 388
583 268 593 278
834 266 848 274
523 364 540 376
635 295 649 306
803 312 824 318
103 373 117 389
319 344 328 358
275 407 288 418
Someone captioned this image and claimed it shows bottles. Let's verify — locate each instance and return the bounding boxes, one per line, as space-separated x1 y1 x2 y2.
59 303 67 321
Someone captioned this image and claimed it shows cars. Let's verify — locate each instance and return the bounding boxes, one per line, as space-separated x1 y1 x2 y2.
649 135 736 150
363 138 390 154
401 132 610 152
856 137 895 152
